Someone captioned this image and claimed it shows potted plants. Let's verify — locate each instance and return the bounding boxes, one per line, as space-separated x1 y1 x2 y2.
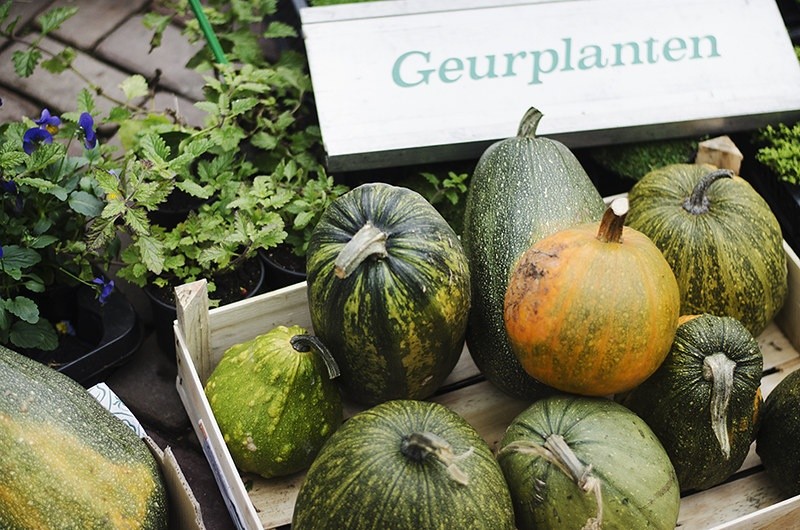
122 59 322 318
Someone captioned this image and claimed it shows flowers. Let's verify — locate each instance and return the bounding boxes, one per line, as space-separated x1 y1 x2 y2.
0 107 116 336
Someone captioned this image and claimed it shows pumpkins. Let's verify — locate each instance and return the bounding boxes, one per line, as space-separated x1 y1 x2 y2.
203 106 800 530
0 345 176 530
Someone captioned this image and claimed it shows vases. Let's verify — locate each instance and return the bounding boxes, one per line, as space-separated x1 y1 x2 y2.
33 264 145 389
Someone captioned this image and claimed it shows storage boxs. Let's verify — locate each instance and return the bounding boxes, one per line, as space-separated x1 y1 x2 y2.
172 136 800 530
